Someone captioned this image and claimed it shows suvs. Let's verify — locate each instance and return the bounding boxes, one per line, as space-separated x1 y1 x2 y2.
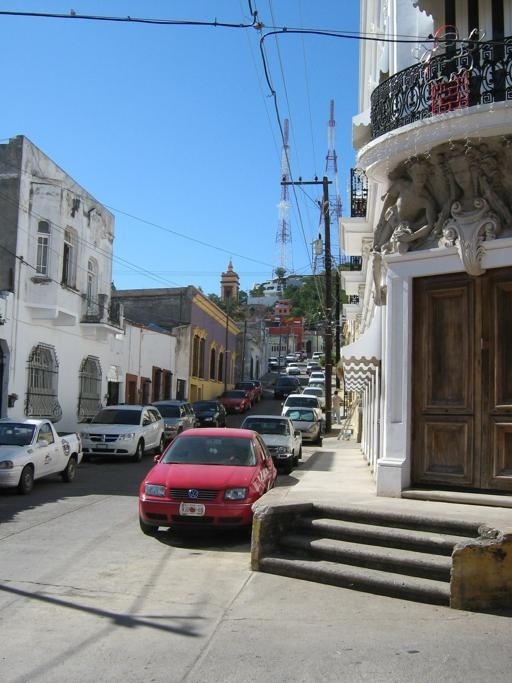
149 399 198 443
81 403 166 463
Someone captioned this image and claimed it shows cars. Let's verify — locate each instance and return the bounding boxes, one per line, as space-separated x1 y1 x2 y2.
137 428 279 535
271 377 302 399
269 349 325 370
250 380 263 397
280 394 323 423
308 371 325 385
287 363 301 375
191 399 227 428
309 366 323 375
302 387 326 409
217 390 251 415
306 362 319 374
240 415 303 468
284 408 323 444
234 381 259 406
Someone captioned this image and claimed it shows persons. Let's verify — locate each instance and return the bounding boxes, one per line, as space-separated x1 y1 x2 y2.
375 160 438 252
447 155 481 212
331 391 343 424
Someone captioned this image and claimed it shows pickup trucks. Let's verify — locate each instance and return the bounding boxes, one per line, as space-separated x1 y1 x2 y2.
0 417 85 497
